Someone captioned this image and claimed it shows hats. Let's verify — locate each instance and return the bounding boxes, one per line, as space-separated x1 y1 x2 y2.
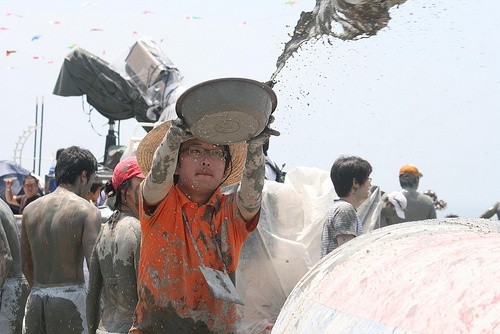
135 119 254 189
112 157 147 190
399 166 424 178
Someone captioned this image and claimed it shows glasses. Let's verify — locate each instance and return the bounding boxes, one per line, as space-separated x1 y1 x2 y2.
178 145 227 160
359 176 373 182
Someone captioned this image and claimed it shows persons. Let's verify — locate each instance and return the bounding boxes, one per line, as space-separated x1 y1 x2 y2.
381 165 437 227
321 156 373 260
128 112 282 334
86 158 147 334
4 174 42 214
263 138 280 182
86 177 104 203
0 198 31 334
45 148 64 195
19 145 103 334
97 178 118 224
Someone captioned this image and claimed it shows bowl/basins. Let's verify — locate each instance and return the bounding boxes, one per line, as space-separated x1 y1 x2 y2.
175 77 278 145
